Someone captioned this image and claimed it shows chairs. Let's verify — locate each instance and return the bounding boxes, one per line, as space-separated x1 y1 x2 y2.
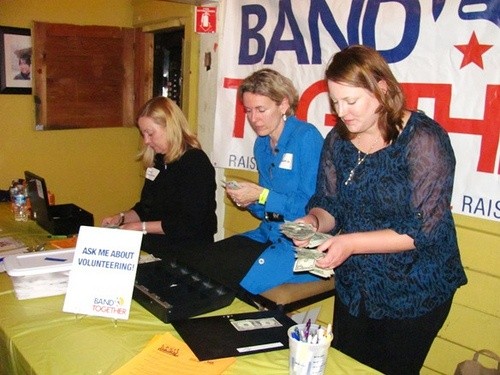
254 274 336 315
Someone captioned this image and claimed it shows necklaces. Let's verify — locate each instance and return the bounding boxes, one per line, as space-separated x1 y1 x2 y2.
345 127 391 184
308 212 320 232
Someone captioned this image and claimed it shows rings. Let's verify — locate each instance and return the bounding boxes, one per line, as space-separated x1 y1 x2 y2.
237 203 241 206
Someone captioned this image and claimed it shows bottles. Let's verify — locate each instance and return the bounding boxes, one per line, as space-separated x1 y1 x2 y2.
46 190 55 208
9 179 38 222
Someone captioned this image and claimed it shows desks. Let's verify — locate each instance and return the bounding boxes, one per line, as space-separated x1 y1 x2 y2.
0 191 390 375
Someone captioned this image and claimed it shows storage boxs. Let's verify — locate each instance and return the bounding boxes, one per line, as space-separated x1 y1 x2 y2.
25 170 95 235
131 256 237 324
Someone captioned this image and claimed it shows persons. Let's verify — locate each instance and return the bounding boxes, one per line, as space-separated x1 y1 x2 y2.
12 54 32 80
101 97 218 243
197 69 324 296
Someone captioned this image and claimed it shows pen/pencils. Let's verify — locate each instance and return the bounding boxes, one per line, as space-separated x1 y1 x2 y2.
290 317 332 375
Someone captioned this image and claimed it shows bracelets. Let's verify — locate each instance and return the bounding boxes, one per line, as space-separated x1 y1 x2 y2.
290 45 470 375
142 221 147 235
258 188 269 206
120 213 124 225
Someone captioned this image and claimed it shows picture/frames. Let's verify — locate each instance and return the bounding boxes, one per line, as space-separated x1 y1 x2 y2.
0 25 32 94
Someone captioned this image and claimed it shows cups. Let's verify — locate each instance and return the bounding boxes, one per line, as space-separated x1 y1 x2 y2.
287 323 334 375
